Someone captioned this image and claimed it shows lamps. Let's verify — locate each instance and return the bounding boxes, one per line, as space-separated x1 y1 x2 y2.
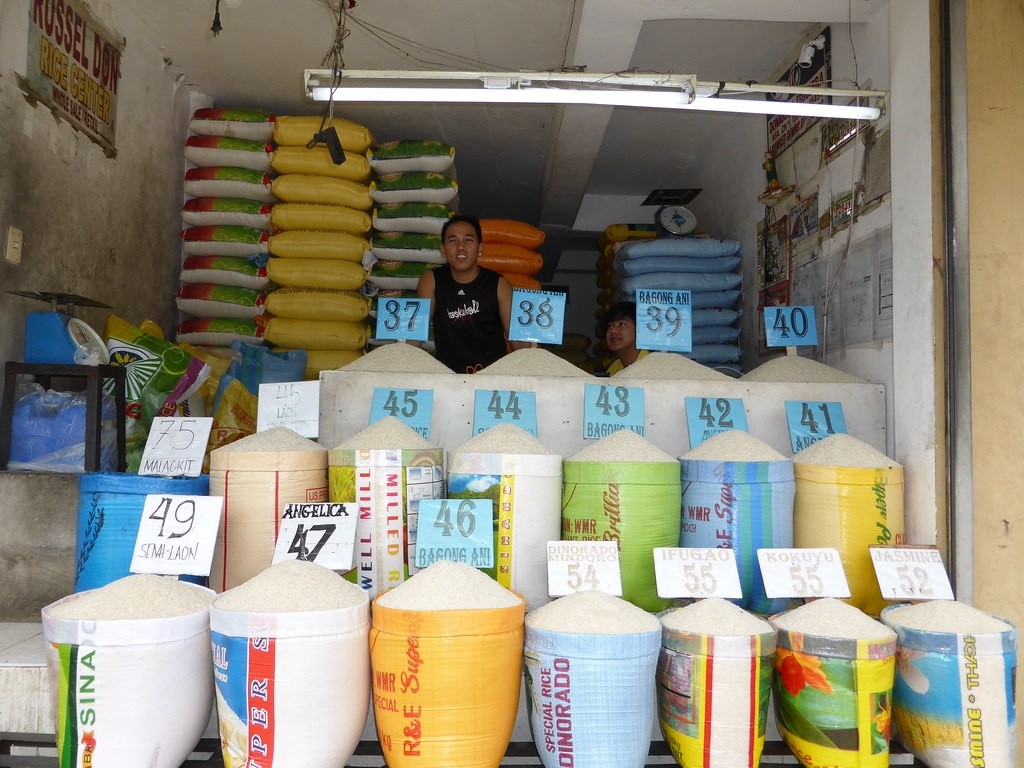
302 67 887 121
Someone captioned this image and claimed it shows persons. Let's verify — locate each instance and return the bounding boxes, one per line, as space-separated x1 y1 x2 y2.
407 215 529 374
604 303 651 375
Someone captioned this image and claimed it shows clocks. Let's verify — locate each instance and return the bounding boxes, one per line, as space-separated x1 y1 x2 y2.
654 202 698 238
